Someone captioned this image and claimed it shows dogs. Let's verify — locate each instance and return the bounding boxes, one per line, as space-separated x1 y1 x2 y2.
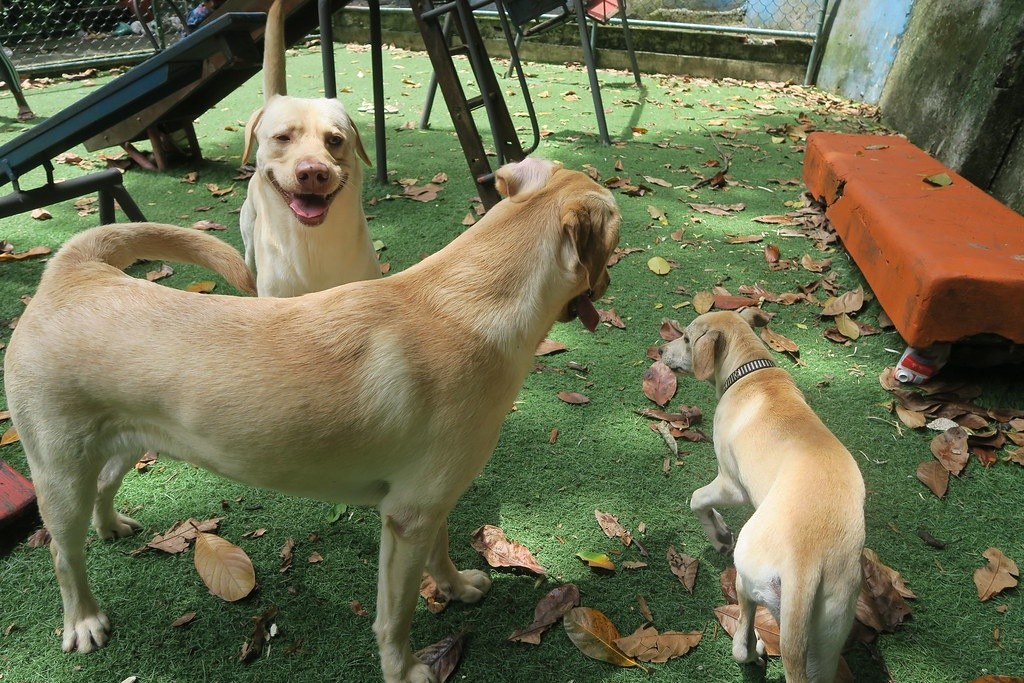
657 306 868 680
239 92 382 299
2 156 622 683
